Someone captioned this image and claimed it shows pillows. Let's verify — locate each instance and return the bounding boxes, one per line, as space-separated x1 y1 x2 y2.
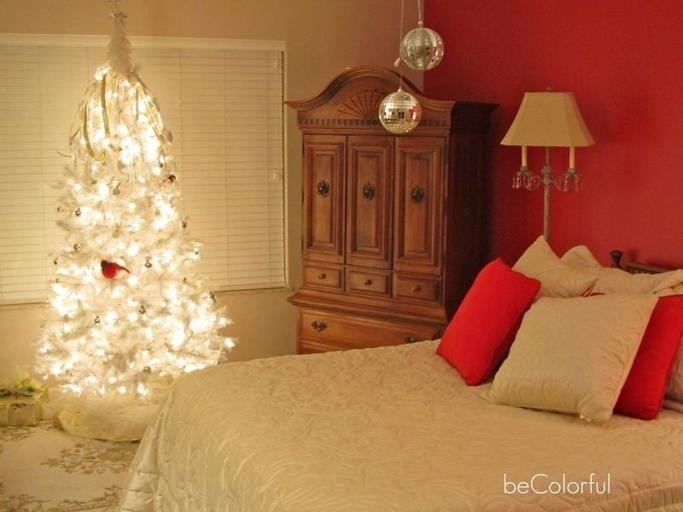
435 233 683 421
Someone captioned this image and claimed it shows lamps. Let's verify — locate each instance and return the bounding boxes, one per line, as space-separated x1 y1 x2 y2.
501 85 596 241
32 0 240 400
380 1 444 135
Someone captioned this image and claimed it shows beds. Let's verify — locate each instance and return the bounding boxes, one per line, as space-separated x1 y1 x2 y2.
171 251 683 510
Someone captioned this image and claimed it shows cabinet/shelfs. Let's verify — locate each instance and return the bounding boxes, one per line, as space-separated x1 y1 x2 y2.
284 67 499 354
